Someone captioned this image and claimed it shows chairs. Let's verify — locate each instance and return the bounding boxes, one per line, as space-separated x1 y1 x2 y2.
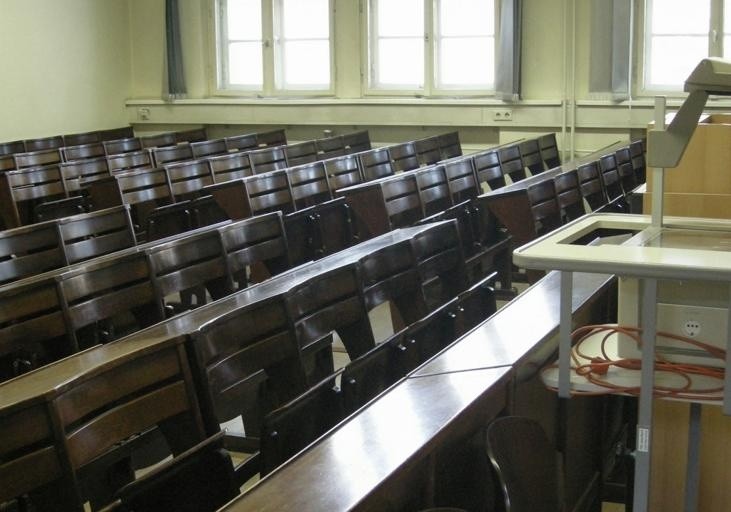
0 125 644 512
